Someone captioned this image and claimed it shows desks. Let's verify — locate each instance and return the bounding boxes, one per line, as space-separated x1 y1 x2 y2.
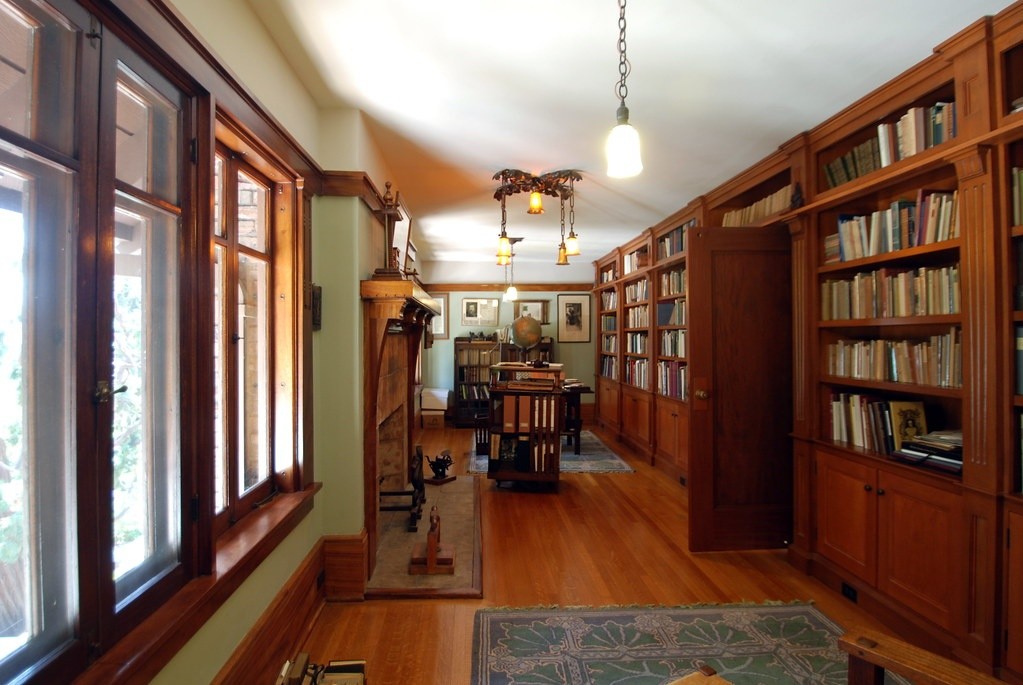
486 387 563 487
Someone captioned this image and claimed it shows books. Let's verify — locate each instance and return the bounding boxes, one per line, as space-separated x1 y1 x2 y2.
600 220 695 400
821 267 962 321
825 102 962 189
830 393 963 476
1011 97 1023 396
722 184 792 227
824 190 960 265
459 349 550 400
828 326 962 388
276 652 366 685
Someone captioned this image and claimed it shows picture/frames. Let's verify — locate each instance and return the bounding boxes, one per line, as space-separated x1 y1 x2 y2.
429 293 449 340
889 399 927 452
513 296 550 325
393 190 414 277
462 298 499 328
556 294 591 343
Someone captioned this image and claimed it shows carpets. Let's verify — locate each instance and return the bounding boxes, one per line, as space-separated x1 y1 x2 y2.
470 600 913 685
467 428 636 476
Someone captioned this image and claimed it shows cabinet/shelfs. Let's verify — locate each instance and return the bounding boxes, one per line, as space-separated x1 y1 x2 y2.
789 26 1023 679
594 214 695 487
455 341 500 403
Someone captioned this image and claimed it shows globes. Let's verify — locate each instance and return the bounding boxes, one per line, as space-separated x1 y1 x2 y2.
512 316 542 364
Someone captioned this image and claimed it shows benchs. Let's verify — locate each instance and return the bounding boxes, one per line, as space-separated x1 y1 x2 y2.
837 624 1009 685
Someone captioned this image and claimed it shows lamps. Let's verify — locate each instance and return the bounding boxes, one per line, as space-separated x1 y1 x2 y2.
605 1 644 179
490 169 582 304
